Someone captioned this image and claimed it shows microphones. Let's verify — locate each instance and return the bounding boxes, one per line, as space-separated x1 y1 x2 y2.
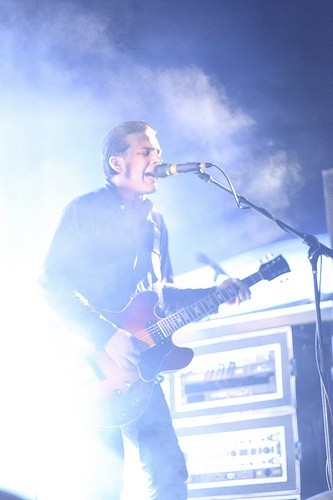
196 253 226 275
154 162 212 178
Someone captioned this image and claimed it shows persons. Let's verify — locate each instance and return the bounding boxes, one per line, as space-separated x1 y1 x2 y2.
40 120 251 500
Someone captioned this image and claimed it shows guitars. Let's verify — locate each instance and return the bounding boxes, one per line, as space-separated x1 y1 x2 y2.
77 252 292 426
